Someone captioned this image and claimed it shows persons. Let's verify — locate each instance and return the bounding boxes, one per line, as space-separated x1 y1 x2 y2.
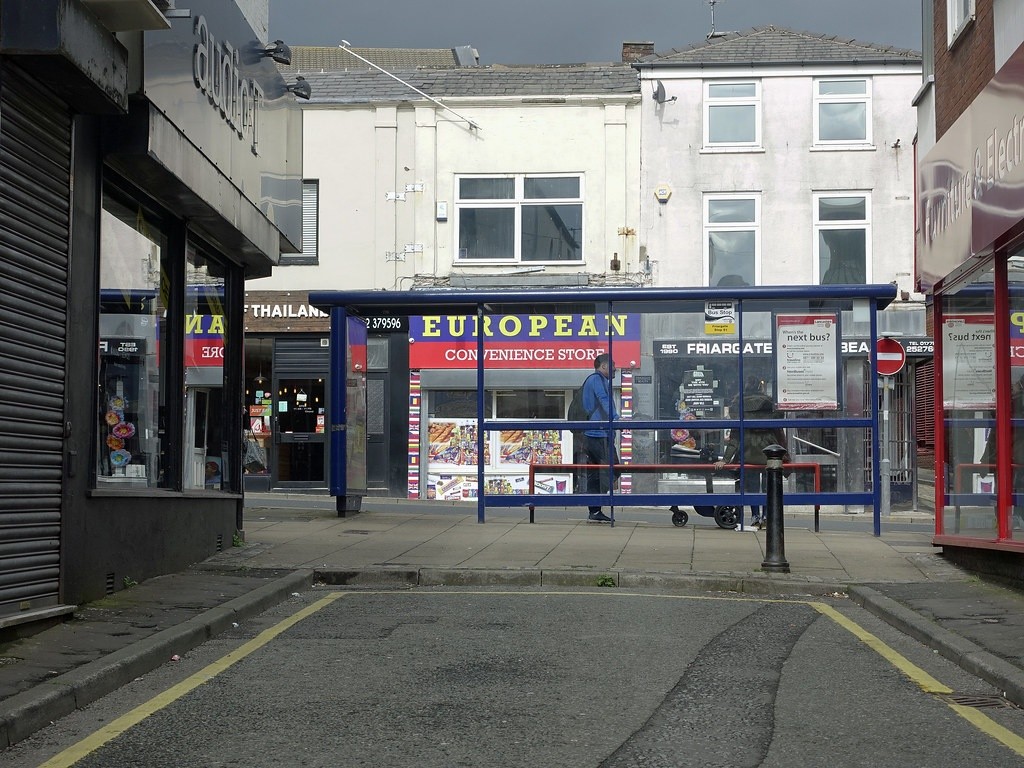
714 375 793 529
981 373 1024 529
582 353 622 523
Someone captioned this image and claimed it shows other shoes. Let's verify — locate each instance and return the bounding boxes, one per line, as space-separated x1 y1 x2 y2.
758 517 768 529
750 515 761 529
1011 516 1021 530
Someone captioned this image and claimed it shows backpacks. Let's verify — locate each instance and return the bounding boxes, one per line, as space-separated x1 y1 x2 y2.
568 372 608 434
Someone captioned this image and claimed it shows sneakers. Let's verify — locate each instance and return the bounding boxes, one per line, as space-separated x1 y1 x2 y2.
588 511 615 523
